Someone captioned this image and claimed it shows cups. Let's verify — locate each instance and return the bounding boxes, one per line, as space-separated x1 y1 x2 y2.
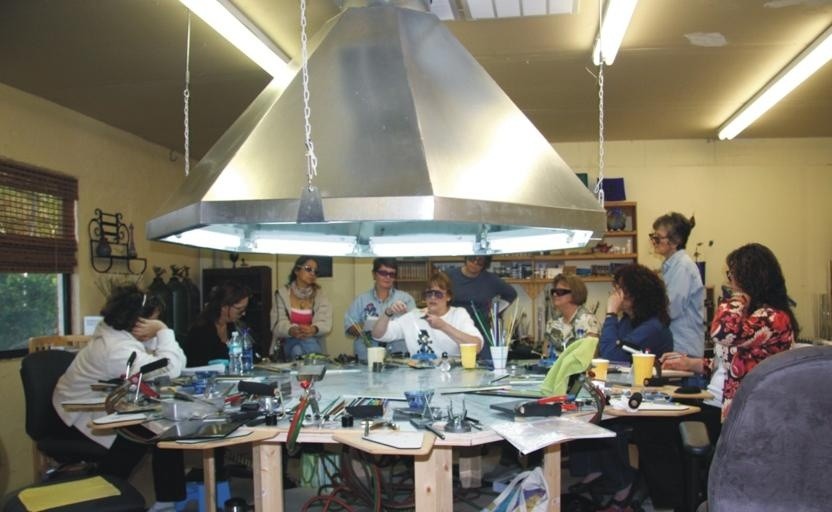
459 343 476 368
489 345 509 369
585 359 610 381
404 390 434 411
366 346 386 371
631 354 656 386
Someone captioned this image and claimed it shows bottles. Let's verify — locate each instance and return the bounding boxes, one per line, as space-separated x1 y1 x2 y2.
273 339 282 362
438 351 451 371
240 325 254 372
227 330 244 376
534 267 544 279
616 239 632 254
492 263 531 280
575 329 584 339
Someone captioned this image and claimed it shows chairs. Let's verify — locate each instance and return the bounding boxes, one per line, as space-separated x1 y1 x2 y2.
17 343 105 475
704 345 831 511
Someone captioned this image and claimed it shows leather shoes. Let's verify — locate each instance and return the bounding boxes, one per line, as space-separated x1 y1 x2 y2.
606 481 636 509
569 474 607 494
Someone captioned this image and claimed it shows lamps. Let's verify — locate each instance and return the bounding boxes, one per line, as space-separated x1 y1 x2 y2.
717 26 830 151
177 0 291 77
591 3 639 66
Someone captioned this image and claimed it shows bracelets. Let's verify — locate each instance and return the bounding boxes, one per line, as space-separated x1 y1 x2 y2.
384 303 394 318
605 311 619 320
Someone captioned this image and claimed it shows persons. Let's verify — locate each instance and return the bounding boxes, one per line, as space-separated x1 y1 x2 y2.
371 269 485 363
269 254 335 362
566 260 674 506
52 283 197 511
649 209 706 358
441 254 519 360
618 243 799 512
186 278 256 370
340 258 419 361
481 271 601 486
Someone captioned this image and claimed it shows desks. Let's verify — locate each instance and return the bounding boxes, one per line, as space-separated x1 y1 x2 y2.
112 352 708 511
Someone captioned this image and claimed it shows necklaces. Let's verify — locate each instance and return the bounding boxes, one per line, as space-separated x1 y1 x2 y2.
297 294 310 309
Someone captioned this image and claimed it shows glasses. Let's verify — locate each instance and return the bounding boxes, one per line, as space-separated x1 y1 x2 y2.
649 233 668 244
296 264 319 275
467 256 487 266
376 270 396 278
423 290 444 300
232 305 246 315
550 289 570 297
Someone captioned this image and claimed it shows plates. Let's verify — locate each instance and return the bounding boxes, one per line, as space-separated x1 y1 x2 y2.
606 207 626 229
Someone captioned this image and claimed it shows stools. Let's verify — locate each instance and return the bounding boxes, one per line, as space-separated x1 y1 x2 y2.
9 471 147 512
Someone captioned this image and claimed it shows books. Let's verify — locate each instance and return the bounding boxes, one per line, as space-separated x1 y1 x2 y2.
491 412 619 457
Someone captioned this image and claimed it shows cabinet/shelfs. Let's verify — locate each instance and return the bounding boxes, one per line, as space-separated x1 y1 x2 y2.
197 264 276 363
387 197 641 335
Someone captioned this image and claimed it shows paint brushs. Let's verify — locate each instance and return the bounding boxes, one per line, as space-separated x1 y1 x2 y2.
348 318 372 348
470 293 526 346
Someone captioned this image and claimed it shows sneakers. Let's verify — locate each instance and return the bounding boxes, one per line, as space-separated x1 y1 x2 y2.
483 464 523 482
